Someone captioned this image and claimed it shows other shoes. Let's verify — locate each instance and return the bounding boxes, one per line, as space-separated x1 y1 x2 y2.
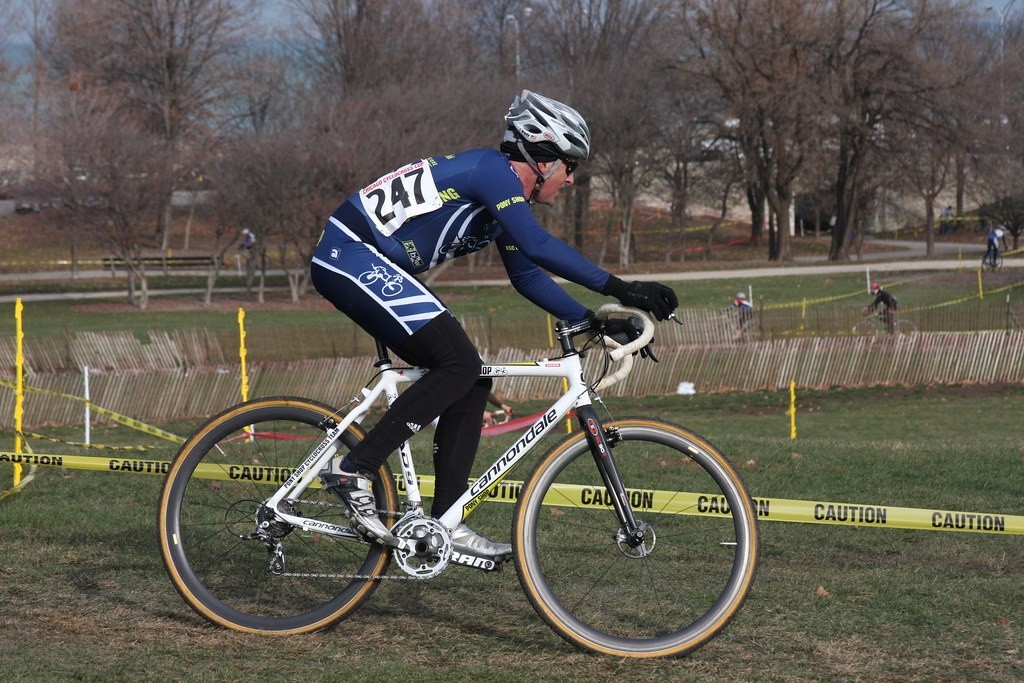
994 263 999 266
982 261 988 264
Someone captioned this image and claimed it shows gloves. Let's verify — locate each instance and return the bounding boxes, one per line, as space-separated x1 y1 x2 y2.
582 309 653 359
601 275 679 322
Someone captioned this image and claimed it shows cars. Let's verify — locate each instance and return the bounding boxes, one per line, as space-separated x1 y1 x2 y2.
0 170 46 198
169 175 217 206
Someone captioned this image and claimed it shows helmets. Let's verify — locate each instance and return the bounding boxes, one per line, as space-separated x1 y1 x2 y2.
871 282 879 289
737 292 745 298
504 88 591 163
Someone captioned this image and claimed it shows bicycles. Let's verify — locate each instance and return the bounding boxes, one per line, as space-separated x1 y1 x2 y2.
853 311 917 345
712 313 765 344
157 307 760 658
981 254 1003 272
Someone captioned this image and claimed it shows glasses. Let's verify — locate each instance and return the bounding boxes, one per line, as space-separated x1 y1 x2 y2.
536 141 580 177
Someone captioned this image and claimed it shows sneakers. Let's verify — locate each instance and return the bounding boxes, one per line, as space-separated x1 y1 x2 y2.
317 454 393 546
451 523 513 559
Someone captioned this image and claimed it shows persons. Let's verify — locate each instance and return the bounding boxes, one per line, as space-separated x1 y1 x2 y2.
862 282 898 334
981 225 1008 267
724 292 754 339
939 205 955 236
308 89 678 565
241 229 256 248
